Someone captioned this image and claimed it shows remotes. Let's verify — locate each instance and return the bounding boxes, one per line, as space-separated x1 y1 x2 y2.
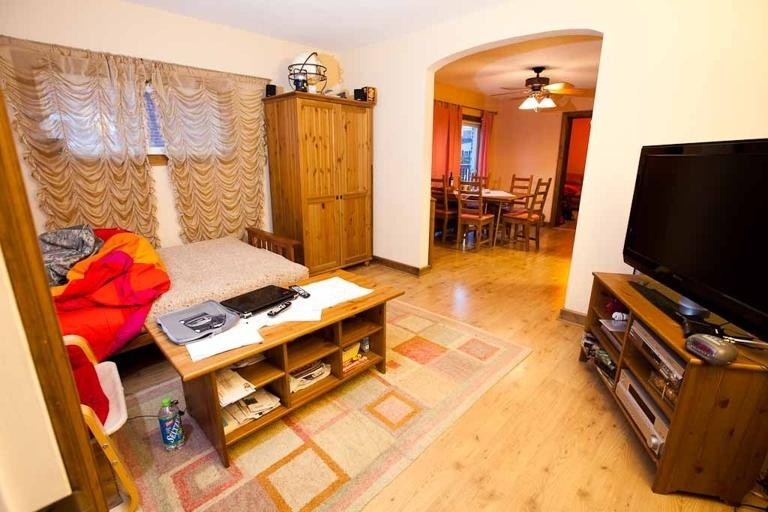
266 301 290 315
288 285 309 298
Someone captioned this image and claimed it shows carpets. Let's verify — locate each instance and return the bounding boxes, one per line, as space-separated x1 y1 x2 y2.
108 300 532 512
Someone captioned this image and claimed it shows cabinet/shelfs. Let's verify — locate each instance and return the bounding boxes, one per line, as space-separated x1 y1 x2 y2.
142 268 406 468
262 91 374 276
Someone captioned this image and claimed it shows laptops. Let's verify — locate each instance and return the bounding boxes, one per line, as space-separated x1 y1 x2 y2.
219 285 298 319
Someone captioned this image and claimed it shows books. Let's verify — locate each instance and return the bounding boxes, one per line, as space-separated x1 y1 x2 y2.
289 359 332 392
221 386 281 435
343 354 368 373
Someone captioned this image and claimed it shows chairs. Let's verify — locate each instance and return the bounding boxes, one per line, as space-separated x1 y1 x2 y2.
62 334 142 512
431 172 552 253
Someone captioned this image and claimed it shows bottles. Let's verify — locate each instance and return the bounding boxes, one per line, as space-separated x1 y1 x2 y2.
158 397 186 451
449 171 454 186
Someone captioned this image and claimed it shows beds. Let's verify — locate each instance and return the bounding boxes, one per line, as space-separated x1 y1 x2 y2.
50 228 310 359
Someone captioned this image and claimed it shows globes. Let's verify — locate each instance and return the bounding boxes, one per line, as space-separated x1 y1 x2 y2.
288 52 327 95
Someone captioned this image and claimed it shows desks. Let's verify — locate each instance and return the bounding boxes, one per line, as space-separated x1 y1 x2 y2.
579 271 768 508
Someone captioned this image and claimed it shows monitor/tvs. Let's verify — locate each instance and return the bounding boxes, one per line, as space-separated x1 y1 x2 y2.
623 138 768 344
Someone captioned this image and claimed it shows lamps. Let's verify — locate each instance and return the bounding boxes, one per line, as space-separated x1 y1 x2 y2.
518 77 557 112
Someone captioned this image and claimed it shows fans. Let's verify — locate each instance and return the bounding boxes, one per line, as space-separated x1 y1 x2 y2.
487 67 575 97
